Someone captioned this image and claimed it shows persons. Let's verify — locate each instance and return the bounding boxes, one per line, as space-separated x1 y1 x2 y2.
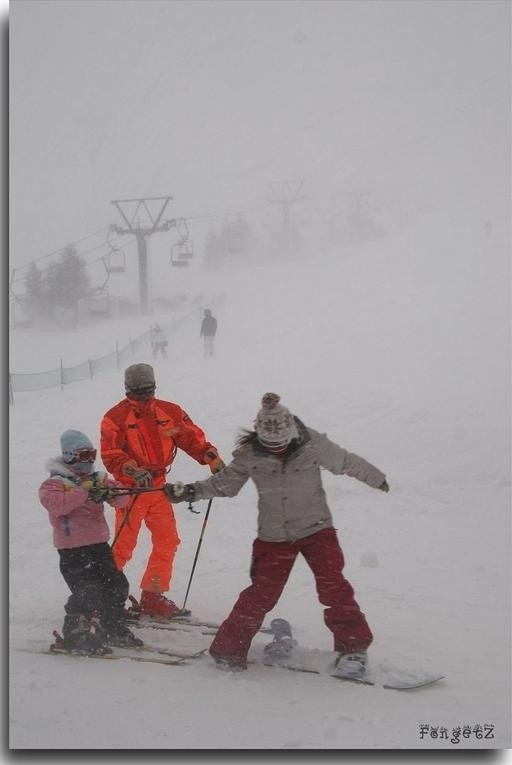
162 393 390 679
100 362 225 618
199 308 217 357
150 324 170 360
38 429 143 653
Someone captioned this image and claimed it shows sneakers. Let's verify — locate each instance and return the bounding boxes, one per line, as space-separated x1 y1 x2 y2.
63 620 102 651
141 591 177 614
215 658 246 670
97 621 143 647
336 650 366 672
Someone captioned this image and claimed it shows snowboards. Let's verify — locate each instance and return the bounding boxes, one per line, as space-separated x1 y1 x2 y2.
210 636 445 689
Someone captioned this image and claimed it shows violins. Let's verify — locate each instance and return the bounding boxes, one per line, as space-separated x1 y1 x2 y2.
13 606 274 665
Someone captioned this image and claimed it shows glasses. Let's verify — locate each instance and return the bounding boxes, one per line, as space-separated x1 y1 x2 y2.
62 448 96 462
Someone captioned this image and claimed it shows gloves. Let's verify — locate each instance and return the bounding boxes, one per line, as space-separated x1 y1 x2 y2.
204 448 225 475
379 480 388 492
123 459 152 487
164 481 195 503
81 471 108 499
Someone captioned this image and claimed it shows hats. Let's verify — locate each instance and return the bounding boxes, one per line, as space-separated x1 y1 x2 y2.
253 393 292 445
60 429 93 473
125 364 154 401
